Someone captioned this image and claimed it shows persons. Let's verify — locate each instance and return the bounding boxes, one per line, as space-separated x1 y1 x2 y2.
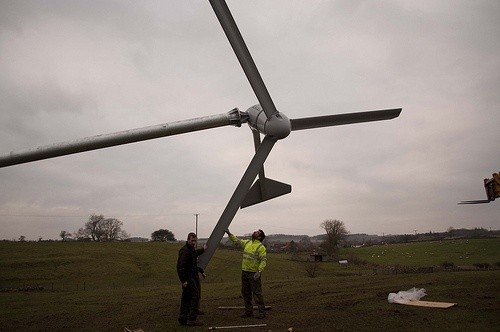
177 232 208 327
224 228 267 319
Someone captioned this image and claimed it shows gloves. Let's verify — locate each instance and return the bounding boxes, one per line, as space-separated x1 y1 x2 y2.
253 270 260 280
223 226 229 233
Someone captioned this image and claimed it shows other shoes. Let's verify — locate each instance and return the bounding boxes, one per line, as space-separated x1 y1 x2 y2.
240 313 254 318
196 309 204 314
255 312 267 318
187 318 204 325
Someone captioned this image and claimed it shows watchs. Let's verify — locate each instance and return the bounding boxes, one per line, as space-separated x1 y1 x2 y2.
258 270 261 273
